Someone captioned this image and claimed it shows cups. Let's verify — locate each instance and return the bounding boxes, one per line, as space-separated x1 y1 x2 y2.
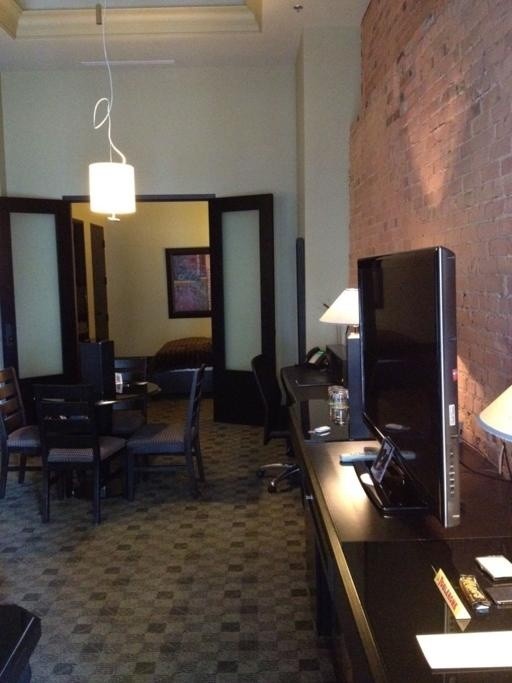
328 385 349 409
330 410 349 426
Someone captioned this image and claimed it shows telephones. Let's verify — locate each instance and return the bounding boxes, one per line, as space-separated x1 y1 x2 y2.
304 347 326 369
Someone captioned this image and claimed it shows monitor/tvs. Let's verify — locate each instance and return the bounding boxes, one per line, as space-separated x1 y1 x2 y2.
353 243 466 530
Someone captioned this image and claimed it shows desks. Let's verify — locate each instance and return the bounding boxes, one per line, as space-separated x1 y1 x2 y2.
279 359 344 400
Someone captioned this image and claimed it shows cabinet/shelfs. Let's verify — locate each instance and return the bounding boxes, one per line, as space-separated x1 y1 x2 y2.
290 399 510 683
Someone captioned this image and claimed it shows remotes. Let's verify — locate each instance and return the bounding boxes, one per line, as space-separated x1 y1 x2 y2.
339 452 381 465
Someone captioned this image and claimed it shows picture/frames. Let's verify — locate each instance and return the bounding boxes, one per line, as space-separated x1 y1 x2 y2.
163 245 212 322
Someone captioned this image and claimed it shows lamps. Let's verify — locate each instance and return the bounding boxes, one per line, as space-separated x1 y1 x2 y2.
319 288 362 336
84 1 139 222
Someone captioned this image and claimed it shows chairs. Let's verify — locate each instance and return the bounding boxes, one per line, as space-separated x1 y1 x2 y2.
252 354 299 494
0 350 205 525
